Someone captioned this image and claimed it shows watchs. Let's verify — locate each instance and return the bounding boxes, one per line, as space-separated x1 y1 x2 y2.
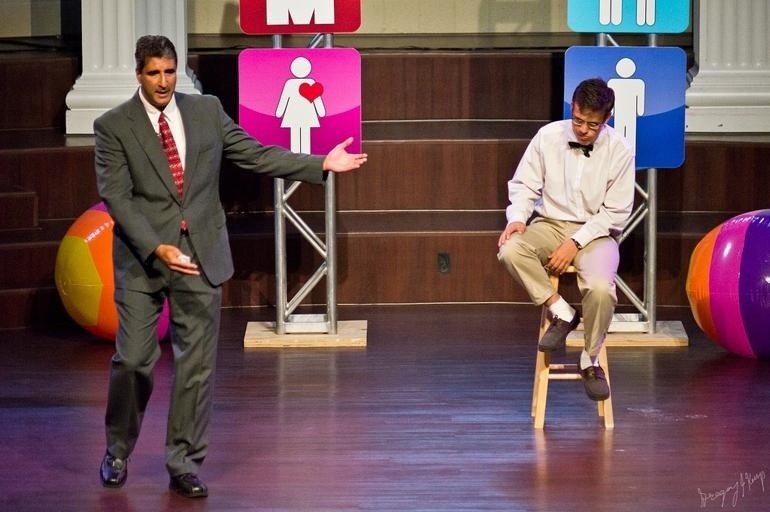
572 237 583 250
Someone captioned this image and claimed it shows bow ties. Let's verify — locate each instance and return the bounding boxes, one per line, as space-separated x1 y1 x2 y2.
568 142 593 151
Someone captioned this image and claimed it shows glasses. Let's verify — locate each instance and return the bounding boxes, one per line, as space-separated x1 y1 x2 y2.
572 108 611 130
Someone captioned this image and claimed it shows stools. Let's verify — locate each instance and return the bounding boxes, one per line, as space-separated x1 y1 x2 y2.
529 262 615 430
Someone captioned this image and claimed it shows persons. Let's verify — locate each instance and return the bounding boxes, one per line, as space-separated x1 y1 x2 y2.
495 75 637 403
89 32 371 504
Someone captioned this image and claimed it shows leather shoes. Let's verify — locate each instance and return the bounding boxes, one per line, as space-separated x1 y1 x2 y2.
577 357 610 401
168 472 209 499
538 305 580 352
100 450 128 488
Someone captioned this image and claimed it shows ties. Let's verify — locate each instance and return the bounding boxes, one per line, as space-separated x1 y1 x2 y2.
155 115 187 231
